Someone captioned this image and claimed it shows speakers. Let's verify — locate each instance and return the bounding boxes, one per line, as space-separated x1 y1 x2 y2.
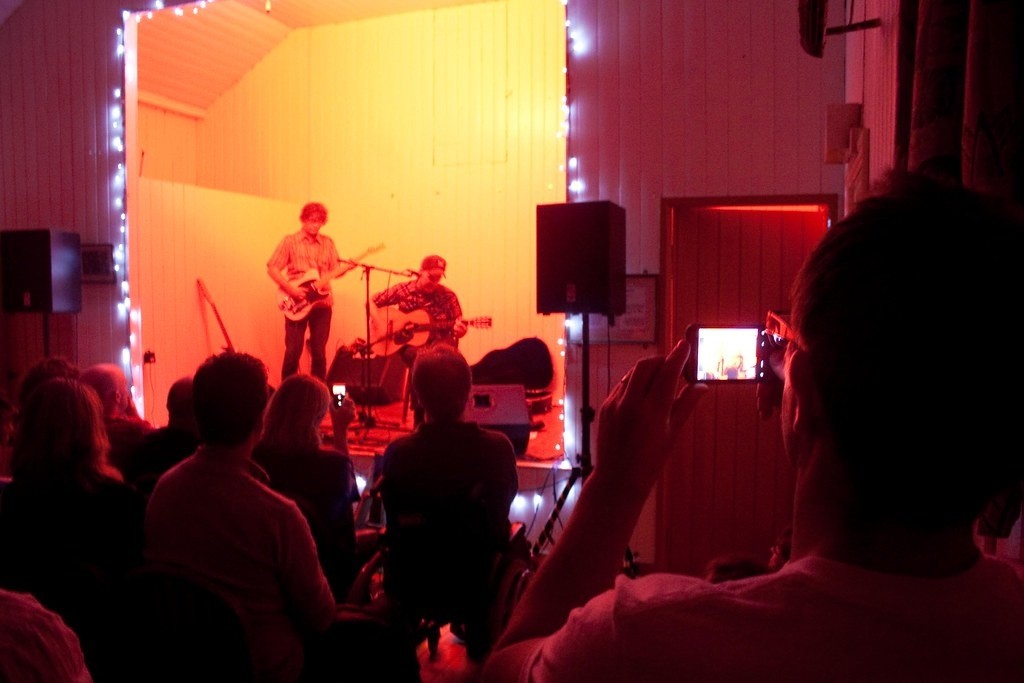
536 199 627 326
0 227 82 315
459 385 530 458
326 345 408 407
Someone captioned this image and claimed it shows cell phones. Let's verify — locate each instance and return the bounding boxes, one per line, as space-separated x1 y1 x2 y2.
685 322 789 384
331 383 346 409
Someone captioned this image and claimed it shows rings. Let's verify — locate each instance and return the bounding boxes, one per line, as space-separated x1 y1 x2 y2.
621 375 629 382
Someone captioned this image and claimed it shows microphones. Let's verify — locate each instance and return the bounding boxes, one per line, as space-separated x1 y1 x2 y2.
406 269 422 278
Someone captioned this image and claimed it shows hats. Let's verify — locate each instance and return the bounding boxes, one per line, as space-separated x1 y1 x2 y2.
421 256 446 279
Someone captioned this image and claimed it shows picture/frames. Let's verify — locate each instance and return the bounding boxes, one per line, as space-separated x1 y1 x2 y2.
81 243 117 286
566 274 659 346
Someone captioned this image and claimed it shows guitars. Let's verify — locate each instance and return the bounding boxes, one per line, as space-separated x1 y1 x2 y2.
370 306 493 358
275 241 385 322
197 277 275 399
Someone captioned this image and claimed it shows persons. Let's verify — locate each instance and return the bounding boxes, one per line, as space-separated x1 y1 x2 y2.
267 203 356 381
368 254 468 368
485 178 1024 683
383 346 518 621
0 351 424 683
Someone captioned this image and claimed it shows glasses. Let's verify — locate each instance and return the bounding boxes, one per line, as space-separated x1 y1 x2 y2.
764 309 826 417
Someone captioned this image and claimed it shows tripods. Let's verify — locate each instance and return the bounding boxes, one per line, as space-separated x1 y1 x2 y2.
517 313 641 605
